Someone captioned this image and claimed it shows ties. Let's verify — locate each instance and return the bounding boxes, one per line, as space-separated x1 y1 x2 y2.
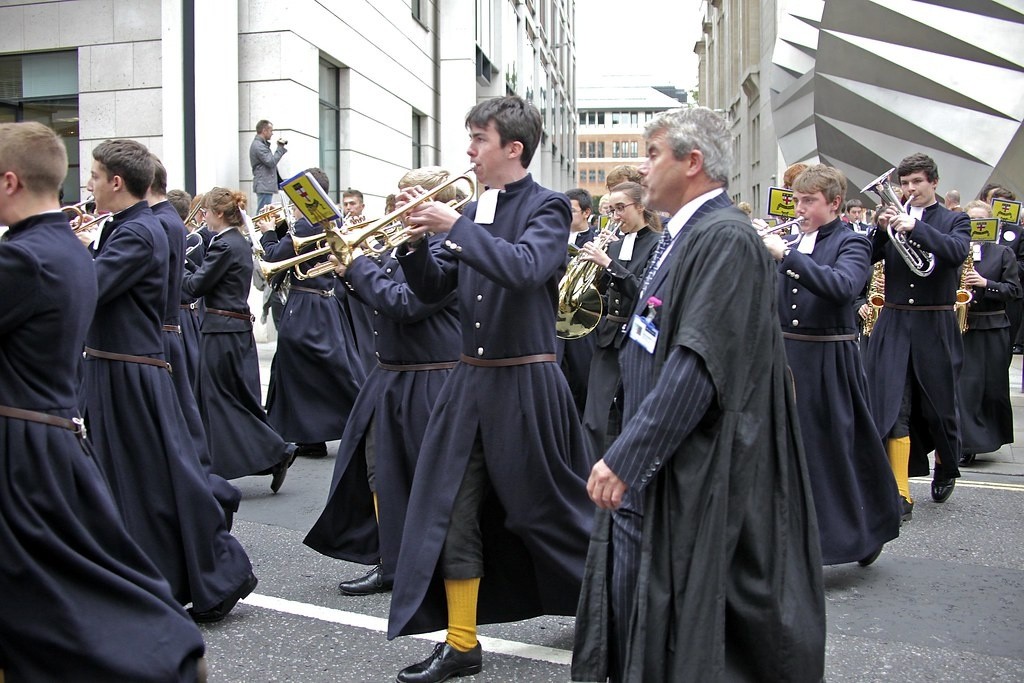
639 227 672 294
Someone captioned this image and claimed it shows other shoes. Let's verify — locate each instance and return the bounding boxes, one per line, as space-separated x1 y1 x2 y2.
301 443 330 460
858 543 884 567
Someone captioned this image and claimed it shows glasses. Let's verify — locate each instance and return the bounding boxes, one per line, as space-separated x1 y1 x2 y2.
605 201 636 218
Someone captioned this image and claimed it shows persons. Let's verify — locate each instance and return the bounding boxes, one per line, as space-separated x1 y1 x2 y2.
250 120 288 230
571 105 828 683
0 123 212 683
841 186 904 374
738 162 808 260
183 187 298 494
561 164 671 423
68 140 258 624
387 96 596 683
981 183 1024 393
257 169 392 459
954 201 1023 466
301 167 471 596
578 182 665 458
867 153 971 527
750 164 901 567
944 190 961 215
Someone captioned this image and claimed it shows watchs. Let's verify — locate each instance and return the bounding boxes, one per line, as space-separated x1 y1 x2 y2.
781 249 791 265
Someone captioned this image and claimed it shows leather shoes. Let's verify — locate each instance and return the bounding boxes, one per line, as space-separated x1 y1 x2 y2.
396 640 482 683
899 494 913 523
269 441 298 493
185 570 259 624
338 563 395 595
959 453 976 466
931 461 956 503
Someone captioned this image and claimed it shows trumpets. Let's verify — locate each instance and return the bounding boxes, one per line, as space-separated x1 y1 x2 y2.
60 184 208 260
759 216 806 257
243 161 479 289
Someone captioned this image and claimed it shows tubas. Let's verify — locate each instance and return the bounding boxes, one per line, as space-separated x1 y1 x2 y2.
554 220 626 340
859 166 936 279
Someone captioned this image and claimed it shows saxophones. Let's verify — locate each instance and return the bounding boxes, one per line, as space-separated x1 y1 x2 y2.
862 259 887 338
954 240 978 335
